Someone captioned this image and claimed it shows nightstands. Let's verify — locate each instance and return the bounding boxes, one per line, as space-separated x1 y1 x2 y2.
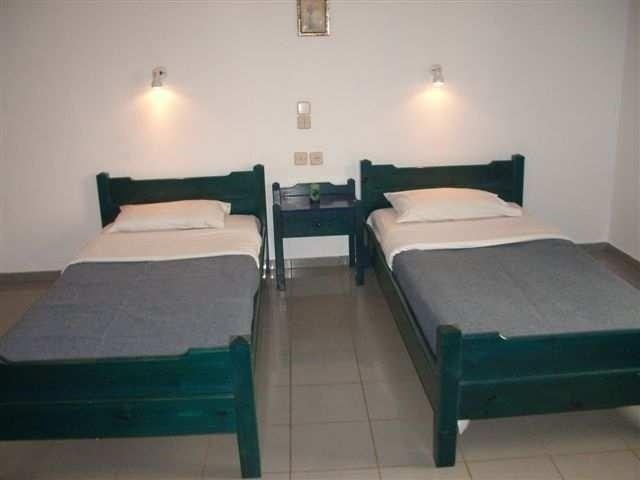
272 179 365 290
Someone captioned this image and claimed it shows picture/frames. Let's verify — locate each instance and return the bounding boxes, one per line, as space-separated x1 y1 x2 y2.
296 0 331 37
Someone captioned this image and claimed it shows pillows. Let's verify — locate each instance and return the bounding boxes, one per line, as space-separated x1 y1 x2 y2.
382 188 521 224
107 200 233 233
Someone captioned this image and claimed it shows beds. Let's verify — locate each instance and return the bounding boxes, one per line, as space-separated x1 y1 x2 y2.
360 152 640 468
0 163 266 479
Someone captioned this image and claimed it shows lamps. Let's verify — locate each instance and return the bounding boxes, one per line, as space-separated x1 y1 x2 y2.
431 64 444 86
150 67 169 89
